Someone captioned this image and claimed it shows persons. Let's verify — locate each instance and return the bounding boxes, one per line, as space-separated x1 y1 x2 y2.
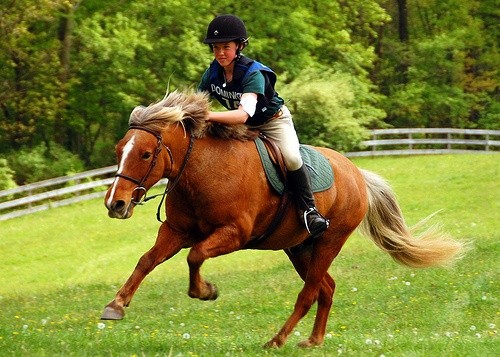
196 14 331 235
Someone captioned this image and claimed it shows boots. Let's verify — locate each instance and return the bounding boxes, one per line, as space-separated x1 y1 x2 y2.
286 161 328 234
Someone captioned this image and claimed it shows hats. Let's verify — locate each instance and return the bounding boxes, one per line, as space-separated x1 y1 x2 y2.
203 15 247 43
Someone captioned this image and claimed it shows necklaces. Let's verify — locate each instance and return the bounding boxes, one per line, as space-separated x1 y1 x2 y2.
220 68 232 87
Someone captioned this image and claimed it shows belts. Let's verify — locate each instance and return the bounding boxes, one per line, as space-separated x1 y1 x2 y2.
253 110 282 126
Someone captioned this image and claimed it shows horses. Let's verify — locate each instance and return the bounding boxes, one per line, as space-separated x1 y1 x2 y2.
101 90 478 350
